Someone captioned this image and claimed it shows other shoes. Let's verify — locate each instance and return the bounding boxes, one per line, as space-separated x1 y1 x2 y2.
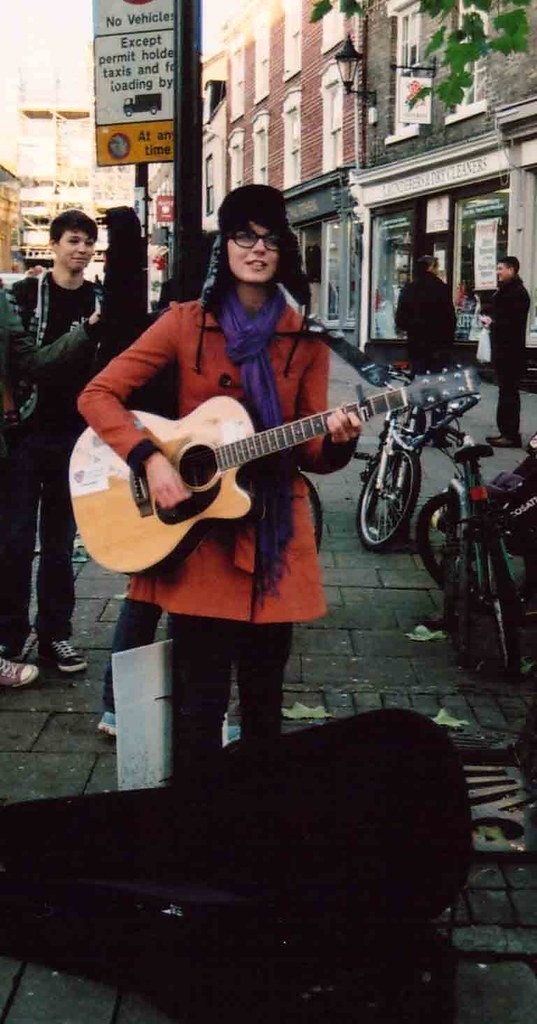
97 711 116 735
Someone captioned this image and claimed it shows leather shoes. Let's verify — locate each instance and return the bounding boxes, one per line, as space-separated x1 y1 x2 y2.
486 435 502 442
491 435 522 447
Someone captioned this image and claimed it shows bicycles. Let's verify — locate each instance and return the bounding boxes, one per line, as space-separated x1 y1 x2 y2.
352 364 466 552
401 394 521 680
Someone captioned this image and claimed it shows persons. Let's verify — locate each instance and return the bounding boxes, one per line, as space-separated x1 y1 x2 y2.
477 256 533 447
96 572 241 750
77 182 366 785
0 267 102 686
392 253 459 448
4 209 101 674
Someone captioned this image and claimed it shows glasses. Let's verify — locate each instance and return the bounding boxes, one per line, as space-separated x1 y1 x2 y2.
228 229 281 251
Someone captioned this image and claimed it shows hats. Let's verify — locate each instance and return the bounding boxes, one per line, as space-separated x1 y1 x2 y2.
218 184 290 234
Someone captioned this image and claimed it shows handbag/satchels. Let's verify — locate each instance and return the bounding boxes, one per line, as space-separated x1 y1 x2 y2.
0 273 50 428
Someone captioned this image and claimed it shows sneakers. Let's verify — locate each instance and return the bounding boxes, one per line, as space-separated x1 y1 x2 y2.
0 643 22 660
0 657 40 688
38 639 88 672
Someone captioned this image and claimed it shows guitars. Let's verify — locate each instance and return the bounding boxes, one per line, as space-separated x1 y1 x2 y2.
66 360 484 578
